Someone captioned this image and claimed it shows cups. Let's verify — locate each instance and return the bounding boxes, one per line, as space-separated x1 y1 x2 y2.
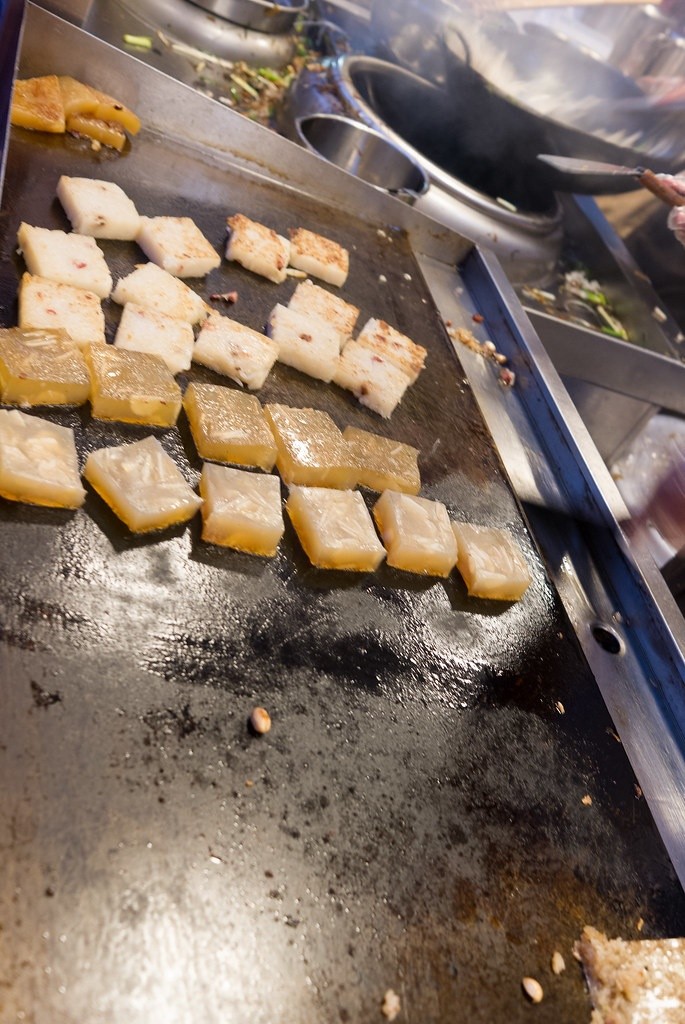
295 113 431 208
609 0 685 83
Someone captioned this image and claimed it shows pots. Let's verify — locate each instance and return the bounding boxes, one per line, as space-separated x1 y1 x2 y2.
438 15 685 198
